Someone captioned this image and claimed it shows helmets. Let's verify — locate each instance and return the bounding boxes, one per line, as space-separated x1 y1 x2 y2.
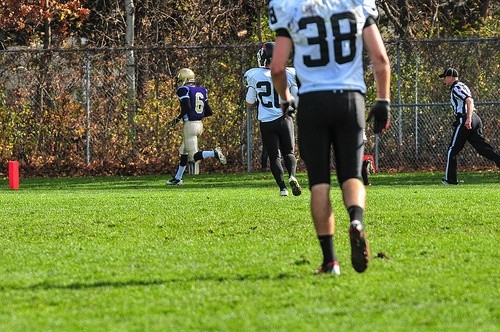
175 68 195 87
260 42 276 66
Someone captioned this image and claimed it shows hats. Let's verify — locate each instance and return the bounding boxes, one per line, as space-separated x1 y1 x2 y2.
438 68 459 78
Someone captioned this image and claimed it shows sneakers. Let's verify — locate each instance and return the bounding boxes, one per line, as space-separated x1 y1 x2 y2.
166 177 183 186
214 148 226 165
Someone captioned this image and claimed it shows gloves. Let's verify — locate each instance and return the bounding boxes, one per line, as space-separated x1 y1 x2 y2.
280 97 298 119
364 99 392 134
171 119 180 125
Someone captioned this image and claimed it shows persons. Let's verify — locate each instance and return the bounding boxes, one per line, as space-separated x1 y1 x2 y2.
267 0 391 279
438 67 500 184
243 42 300 196
165 67 227 185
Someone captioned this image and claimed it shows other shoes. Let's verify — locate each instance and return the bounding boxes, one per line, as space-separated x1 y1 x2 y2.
441 177 459 186
288 176 302 197
315 260 340 276
280 188 288 196
349 221 369 273
